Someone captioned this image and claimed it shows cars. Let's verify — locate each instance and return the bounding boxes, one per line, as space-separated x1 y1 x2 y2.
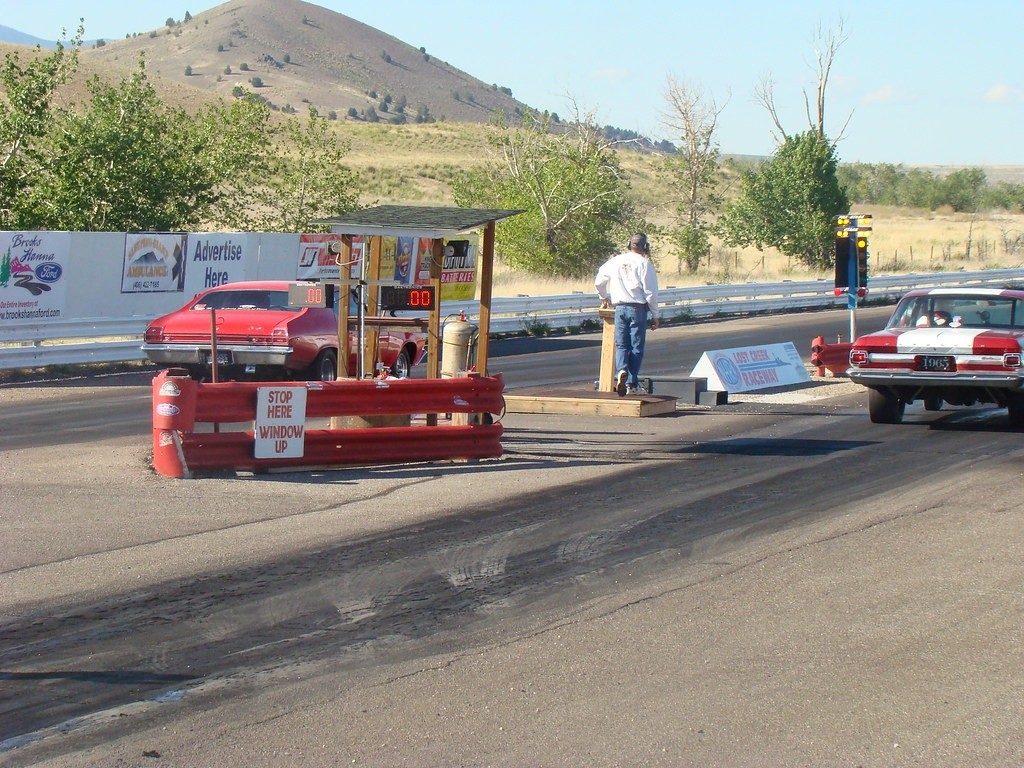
141 280 425 382
846 286 1024 425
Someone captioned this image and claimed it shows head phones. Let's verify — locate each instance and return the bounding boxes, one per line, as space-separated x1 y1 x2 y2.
627 239 650 251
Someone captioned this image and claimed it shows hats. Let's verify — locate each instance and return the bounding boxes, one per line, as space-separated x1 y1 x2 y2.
631 234 647 249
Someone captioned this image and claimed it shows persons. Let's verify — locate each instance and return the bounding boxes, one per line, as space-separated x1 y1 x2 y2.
594 233 659 397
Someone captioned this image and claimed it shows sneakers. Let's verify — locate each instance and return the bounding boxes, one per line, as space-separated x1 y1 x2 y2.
617 369 628 397
626 383 647 394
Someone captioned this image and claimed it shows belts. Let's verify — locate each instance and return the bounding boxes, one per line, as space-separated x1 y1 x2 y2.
620 303 645 308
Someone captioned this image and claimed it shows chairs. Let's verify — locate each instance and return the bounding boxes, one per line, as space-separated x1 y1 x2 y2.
934 311 952 325
238 294 270 308
